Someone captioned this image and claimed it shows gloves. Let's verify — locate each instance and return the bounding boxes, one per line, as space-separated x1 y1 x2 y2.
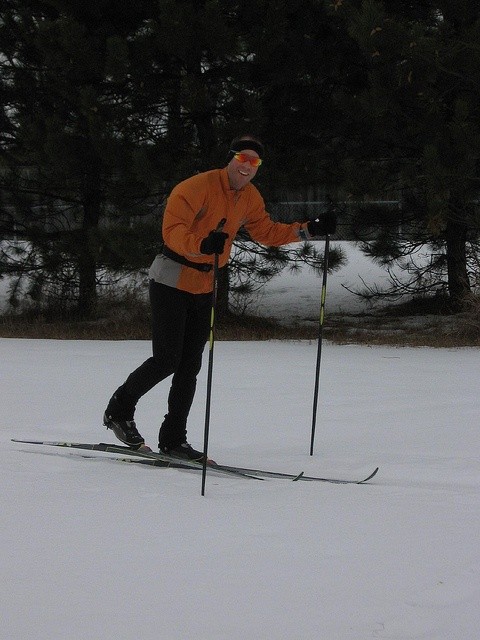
200 229 229 255
307 210 336 237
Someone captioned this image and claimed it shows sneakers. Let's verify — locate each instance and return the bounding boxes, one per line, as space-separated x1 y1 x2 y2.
158 441 204 462
103 413 144 446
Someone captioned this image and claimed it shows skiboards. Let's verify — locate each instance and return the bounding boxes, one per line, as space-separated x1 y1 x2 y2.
9 435 383 489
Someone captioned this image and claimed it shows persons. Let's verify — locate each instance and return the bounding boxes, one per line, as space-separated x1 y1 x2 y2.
102 135 336 462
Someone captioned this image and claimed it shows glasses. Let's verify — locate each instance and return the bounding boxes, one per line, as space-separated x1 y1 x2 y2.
228 150 264 166
